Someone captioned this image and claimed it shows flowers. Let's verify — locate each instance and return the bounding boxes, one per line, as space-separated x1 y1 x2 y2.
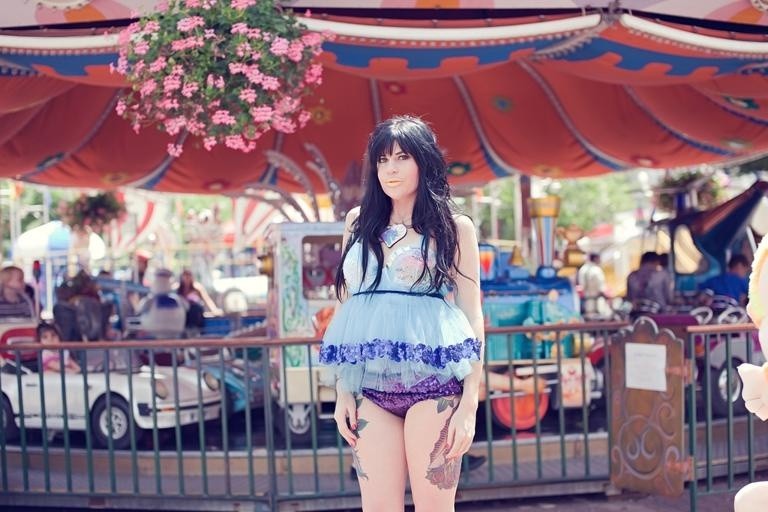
106 0 324 157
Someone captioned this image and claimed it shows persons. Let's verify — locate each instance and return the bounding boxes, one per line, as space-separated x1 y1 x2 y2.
37 322 82 374
626 251 675 317
0 266 31 318
334 116 486 512
578 252 614 318
698 254 750 307
177 268 217 313
